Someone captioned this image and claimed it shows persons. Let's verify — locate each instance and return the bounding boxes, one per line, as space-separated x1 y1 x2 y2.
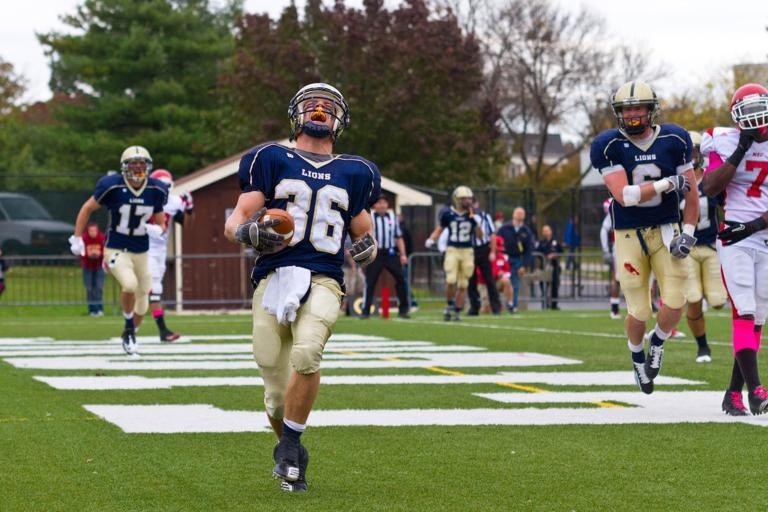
82 225 108 316
590 80 698 395
364 196 412 319
700 84 768 416
223 85 382 492
425 186 483 322
537 223 563 311
467 198 502 316
600 198 686 339
497 206 535 312
147 169 195 343
394 216 419 314
680 130 726 362
70 146 168 354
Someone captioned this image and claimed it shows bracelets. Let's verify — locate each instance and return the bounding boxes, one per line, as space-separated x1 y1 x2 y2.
652 179 669 194
683 223 695 237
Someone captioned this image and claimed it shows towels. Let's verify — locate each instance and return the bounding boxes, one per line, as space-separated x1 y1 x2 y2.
262 265 312 325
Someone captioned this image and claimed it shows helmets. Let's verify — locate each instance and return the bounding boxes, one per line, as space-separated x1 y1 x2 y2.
686 131 703 147
149 169 173 186
451 186 475 199
119 146 153 164
700 126 738 157
612 80 657 108
287 82 348 112
729 83 768 111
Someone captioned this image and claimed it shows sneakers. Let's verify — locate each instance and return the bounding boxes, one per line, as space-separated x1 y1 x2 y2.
609 309 621 319
644 329 664 379
747 384 768 414
281 443 309 492
122 326 139 360
160 328 180 342
360 298 561 321
273 440 300 482
695 345 711 362
670 330 687 339
632 361 654 394
721 390 749 416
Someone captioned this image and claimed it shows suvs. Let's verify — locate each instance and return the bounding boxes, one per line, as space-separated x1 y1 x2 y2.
0 189 76 266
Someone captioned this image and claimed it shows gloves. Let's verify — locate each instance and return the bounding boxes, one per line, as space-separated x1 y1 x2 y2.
716 217 766 247
424 239 435 248
349 231 379 267
669 233 698 260
68 235 86 257
142 222 164 239
473 214 482 227
727 127 761 168
179 190 194 210
234 206 284 254
663 173 691 194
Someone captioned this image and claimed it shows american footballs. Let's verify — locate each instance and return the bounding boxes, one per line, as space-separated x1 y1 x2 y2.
258 208 295 251
